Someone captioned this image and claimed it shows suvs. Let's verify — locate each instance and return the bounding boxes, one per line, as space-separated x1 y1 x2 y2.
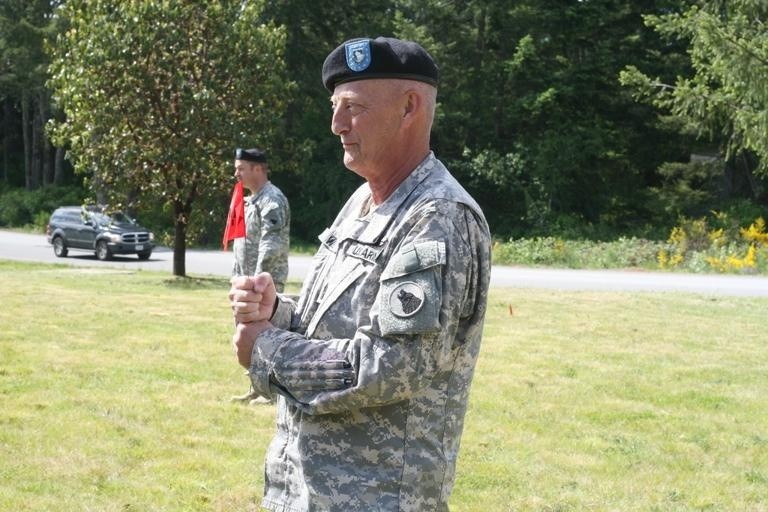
43 202 157 264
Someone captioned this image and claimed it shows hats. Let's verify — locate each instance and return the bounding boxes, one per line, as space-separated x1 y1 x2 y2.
234 147 267 163
322 34 440 92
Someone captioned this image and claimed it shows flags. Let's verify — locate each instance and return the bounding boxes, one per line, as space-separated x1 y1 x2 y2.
222 183 245 249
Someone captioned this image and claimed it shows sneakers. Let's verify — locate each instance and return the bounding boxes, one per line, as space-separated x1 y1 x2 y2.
232 389 256 402
250 393 273 405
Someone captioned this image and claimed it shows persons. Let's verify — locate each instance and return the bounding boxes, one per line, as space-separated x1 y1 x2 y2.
231 148 291 406
228 37 492 512
